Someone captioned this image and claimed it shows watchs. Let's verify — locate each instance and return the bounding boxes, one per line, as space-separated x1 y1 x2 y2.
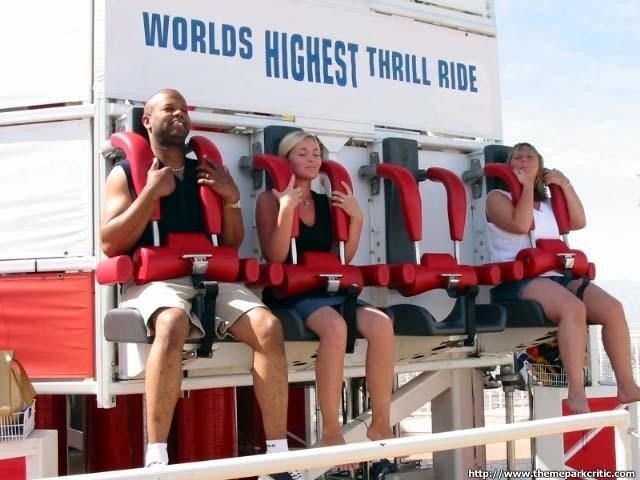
223 200 243 209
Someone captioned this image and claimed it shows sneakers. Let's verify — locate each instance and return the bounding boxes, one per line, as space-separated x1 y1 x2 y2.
258 469 304 480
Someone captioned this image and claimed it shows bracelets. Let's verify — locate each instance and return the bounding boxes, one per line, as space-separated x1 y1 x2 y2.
563 182 572 190
302 199 313 208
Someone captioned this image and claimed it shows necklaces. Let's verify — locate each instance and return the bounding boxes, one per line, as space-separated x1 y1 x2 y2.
169 167 184 173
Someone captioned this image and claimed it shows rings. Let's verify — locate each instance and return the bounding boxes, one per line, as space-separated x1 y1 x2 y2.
340 199 343 204
205 173 210 178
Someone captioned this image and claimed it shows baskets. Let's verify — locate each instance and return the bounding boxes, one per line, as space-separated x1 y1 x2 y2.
524 363 588 388
0 399 36 444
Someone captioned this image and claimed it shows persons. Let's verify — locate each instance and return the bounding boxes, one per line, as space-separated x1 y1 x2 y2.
256 132 409 473
486 141 640 415
100 90 290 467
527 345 558 381
